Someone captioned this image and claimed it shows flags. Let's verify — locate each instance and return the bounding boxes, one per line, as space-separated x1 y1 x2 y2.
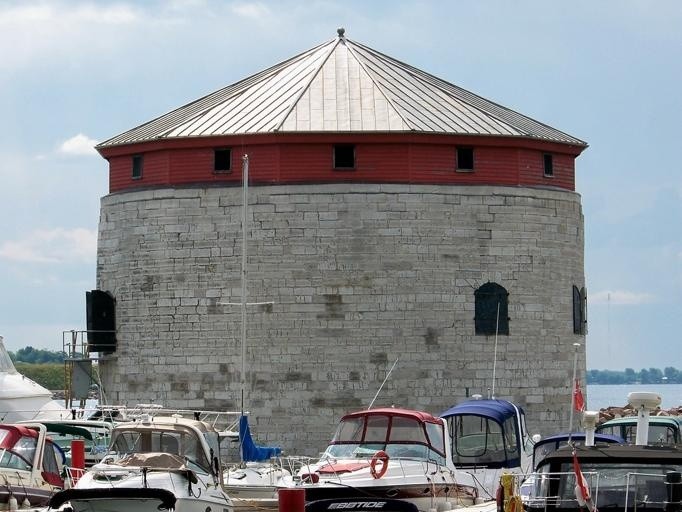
576 382 586 413
571 456 590 503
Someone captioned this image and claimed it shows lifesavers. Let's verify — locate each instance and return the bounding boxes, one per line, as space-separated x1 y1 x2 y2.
370 451 388 479
